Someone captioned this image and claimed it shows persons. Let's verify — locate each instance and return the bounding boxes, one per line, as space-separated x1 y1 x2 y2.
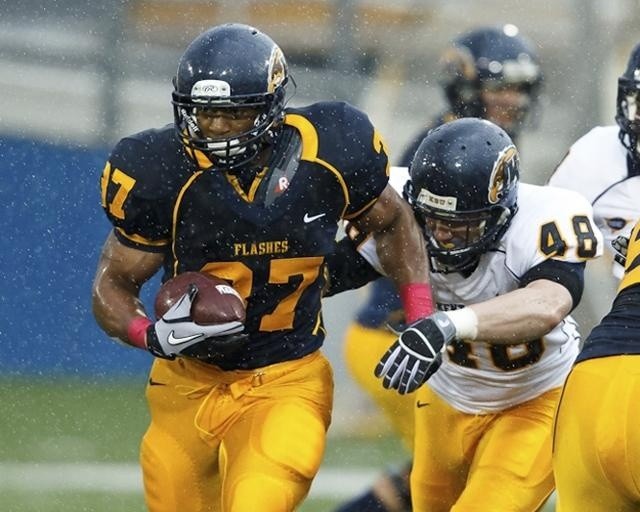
328 30 541 511
317 117 604 512
91 24 434 512
550 223 639 512
540 42 639 511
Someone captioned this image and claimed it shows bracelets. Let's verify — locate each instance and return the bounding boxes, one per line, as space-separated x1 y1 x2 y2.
448 307 477 340
401 282 431 322
125 315 148 348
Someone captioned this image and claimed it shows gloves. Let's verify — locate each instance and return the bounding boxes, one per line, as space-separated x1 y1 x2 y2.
373 310 457 395
611 235 630 268
146 281 245 362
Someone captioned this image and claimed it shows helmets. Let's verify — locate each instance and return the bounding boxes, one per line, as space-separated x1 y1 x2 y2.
613 41 639 167
401 116 520 275
170 22 297 173
435 27 543 139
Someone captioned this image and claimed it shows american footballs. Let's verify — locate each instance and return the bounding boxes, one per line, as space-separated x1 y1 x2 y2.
154 272 245 326
